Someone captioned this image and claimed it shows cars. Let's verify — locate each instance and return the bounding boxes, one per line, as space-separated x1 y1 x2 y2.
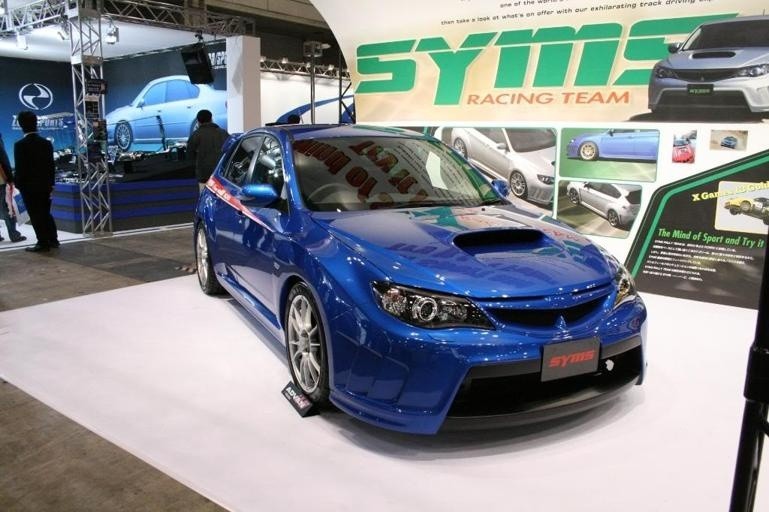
194 122 647 435
725 195 768 224
648 17 768 115
721 135 737 148
672 133 694 162
565 128 659 163
432 125 555 207
566 180 641 227
105 75 226 152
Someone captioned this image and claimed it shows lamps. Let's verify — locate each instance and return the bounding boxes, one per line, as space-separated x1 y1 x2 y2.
104 17 120 45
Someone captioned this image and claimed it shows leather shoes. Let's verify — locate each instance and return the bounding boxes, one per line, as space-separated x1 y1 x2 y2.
25 238 60 253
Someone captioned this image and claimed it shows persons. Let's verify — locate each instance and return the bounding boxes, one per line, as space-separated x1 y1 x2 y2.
185 108 231 197
0 133 27 244
13 110 61 253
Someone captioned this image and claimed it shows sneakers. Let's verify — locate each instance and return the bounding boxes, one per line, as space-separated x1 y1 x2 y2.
12 235 26 243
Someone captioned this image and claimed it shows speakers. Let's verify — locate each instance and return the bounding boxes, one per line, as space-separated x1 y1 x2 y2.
181 43 216 84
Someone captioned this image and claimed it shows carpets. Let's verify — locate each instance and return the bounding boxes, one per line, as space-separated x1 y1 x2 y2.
0 271 769 510
